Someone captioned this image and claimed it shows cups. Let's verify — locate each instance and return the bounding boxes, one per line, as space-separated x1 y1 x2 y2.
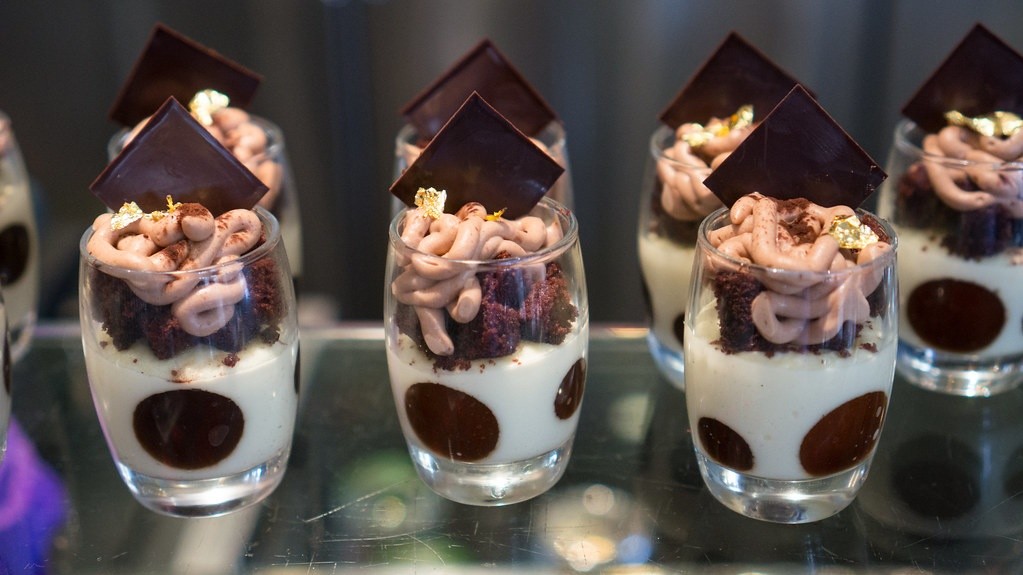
385 197 590 507
78 206 300 518
635 124 727 391
108 113 301 301
1 116 39 463
876 118 1023 398
391 119 573 210
682 205 899 524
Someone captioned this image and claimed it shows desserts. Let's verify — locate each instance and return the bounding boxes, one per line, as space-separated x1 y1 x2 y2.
75 22 1023 523
